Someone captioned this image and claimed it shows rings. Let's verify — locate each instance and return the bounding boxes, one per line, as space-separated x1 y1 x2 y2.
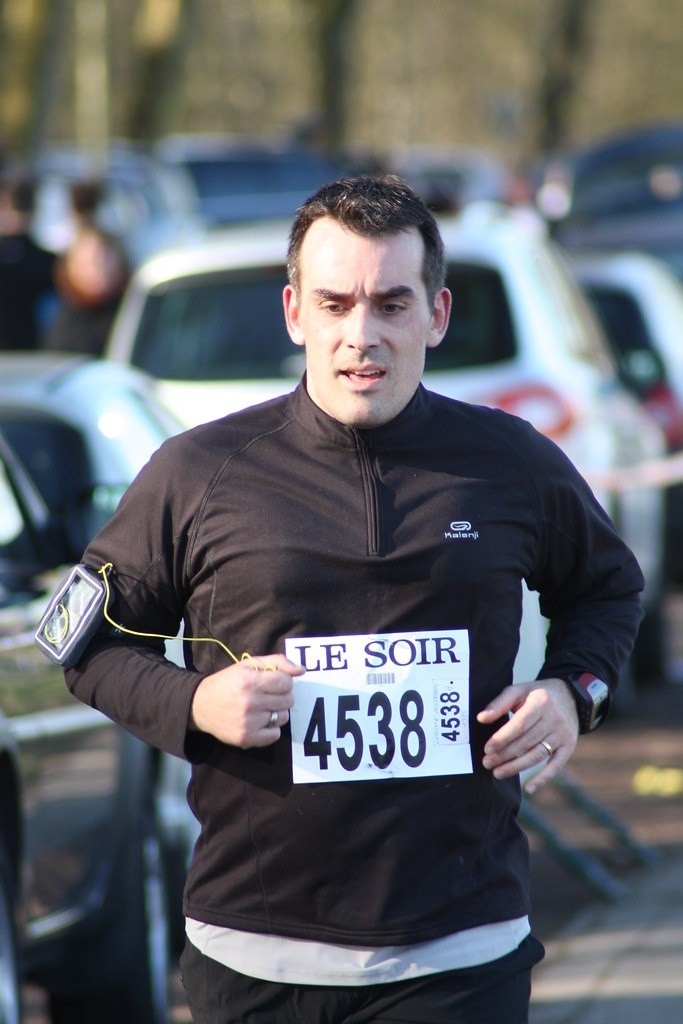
267 711 278 727
539 741 553 755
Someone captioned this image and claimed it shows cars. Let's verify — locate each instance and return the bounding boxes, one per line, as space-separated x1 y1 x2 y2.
11 126 683 588
0 436 197 1023
0 349 191 667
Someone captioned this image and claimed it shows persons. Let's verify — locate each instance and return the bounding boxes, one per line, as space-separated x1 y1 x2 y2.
62 175 645 1024
0 169 129 355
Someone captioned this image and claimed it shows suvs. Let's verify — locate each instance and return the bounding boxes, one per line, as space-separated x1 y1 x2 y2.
108 199 674 720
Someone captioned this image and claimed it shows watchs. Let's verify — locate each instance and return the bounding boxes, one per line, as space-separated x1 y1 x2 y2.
567 672 609 734
